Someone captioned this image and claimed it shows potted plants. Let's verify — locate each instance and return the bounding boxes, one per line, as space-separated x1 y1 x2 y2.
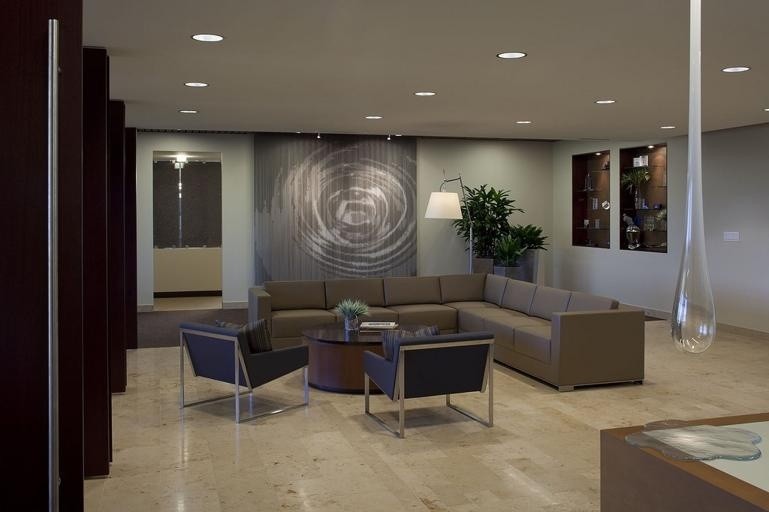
452 184 553 283
333 295 369 330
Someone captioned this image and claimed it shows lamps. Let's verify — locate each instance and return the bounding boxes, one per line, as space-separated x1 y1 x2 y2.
422 174 476 276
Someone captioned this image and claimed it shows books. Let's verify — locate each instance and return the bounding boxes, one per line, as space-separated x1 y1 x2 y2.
359 321 400 333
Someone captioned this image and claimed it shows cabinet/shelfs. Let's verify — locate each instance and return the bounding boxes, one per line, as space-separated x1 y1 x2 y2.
572 143 666 255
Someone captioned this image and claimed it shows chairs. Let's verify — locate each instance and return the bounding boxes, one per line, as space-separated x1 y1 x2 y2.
176 323 311 424
352 327 499 438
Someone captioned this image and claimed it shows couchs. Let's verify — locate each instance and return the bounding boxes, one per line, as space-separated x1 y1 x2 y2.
244 274 646 395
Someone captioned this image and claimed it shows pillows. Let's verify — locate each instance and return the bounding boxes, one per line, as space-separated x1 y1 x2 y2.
216 319 274 353
379 324 440 362
